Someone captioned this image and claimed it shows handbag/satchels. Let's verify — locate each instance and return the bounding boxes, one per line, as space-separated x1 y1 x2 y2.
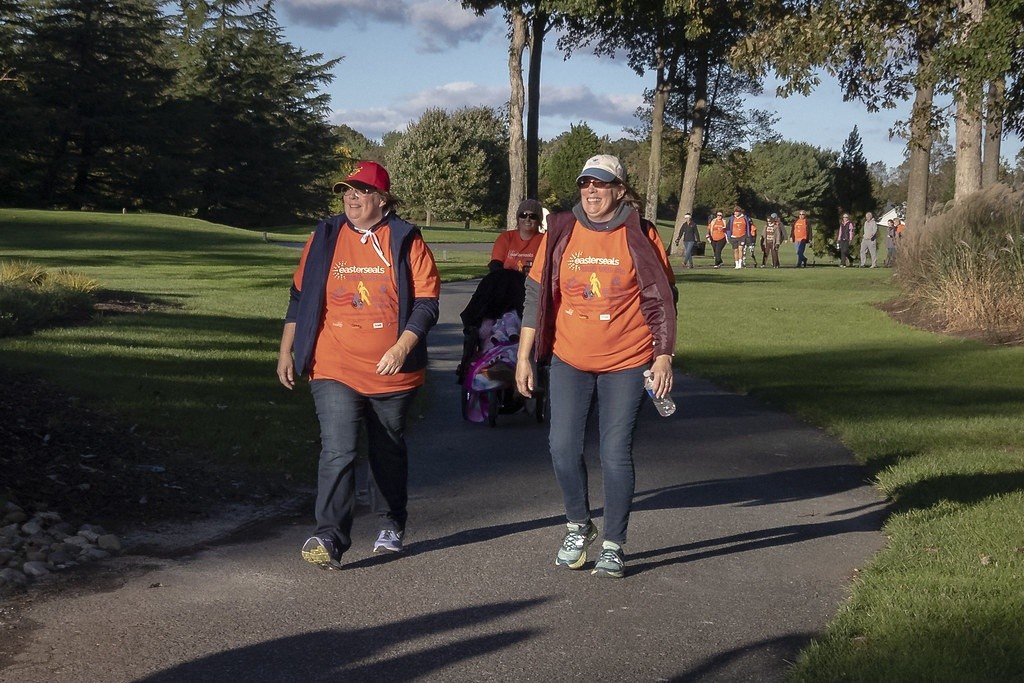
690 241 706 256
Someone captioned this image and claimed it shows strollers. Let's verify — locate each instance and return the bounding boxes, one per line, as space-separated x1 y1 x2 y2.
457 268 553 425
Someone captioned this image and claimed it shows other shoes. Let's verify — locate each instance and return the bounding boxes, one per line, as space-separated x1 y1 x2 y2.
869 264 878 268
857 265 866 268
840 264 846 268
679 258 808 270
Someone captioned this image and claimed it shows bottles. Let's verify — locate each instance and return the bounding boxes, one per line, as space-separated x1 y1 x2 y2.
643 370 676 417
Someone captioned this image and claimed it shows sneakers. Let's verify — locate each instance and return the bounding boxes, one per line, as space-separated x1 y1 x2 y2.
555 518 598 570
372 528 403 554
301 537 342 572
590 540 625 578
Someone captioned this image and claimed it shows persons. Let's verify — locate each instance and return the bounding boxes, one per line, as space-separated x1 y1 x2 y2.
790 210 812 267
861 213 878 268
641 219 678 305
760 214 788 268
886 218 905 267
675 213 700 269
489 200 544 411
276 162 440 569
516 154 679 579
706 206 757 269
837 214 854 267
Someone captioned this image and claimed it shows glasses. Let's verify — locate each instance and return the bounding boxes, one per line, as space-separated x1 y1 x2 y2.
520 211 538 221
799 213 805 216
576 177 620 189
842 217 848 218
717 215 722 216
340 186 379 200
767 221 771 222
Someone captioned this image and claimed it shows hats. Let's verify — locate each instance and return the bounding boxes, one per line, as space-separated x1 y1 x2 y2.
334 160 391 196
575 155 625 184
516 199 542 225
